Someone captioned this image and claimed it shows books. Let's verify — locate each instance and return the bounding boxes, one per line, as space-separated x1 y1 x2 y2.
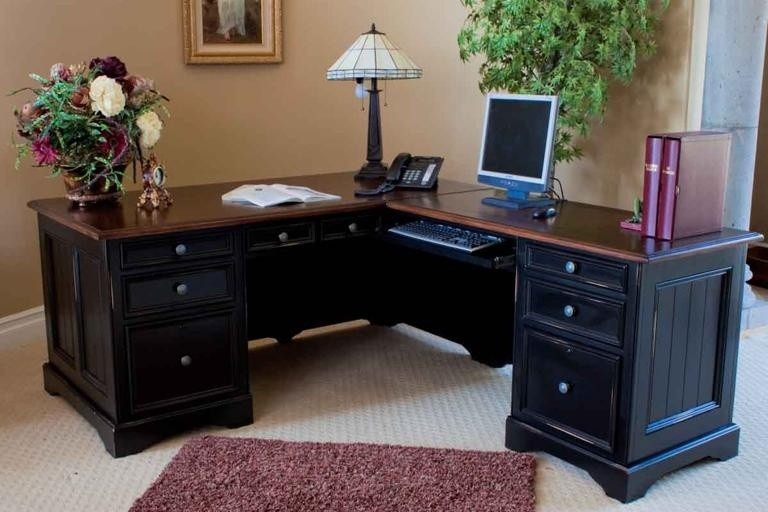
639 130 724 237
221 182 342 208
655 124 732 241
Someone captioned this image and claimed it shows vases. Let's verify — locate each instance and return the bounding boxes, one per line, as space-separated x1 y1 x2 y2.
62 171 125 207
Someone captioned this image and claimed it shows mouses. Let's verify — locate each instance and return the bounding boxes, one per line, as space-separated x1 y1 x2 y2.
532 207 557 219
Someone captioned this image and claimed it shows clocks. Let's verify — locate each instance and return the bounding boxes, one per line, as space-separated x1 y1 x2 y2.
137 153 173 209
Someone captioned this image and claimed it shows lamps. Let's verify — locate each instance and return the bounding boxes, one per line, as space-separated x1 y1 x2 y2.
327 24 423 181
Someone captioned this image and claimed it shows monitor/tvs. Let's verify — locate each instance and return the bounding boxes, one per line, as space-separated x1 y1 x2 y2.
476 94 560 210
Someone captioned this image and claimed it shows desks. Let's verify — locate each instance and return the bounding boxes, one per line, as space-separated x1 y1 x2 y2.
28 172 764 503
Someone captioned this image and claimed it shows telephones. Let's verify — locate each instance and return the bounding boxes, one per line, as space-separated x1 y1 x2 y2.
385 153 444 191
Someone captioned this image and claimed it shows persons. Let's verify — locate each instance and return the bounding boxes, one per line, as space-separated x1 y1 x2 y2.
216 1 248 40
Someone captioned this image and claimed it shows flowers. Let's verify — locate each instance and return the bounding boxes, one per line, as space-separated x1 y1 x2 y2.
5 56 172 197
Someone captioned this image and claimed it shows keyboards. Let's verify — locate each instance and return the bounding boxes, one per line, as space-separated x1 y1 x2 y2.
388 219 504 255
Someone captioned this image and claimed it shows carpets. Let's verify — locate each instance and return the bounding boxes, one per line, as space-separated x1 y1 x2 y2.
128 435 535 512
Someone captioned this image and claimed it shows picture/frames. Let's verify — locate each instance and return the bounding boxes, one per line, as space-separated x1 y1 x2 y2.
182 0 282 66
